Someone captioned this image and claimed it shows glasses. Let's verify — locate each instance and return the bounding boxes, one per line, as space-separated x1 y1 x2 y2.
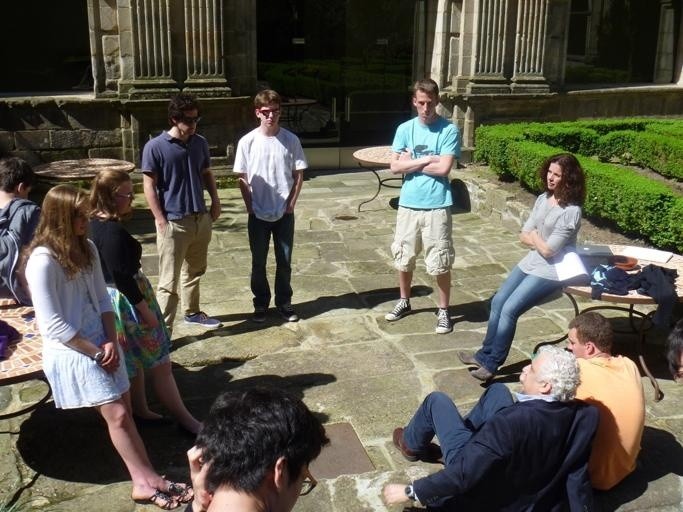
183 117 202 122
299 464 318 497
259 108 280 116
117 190 132 198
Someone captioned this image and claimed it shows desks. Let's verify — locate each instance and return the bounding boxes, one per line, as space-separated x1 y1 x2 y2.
532 245 682 401
280 97 317 133
0 297 50 419
352 146 400 212
34 156 135 181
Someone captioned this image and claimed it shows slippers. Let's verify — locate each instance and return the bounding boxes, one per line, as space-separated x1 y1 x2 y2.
131 473 193 510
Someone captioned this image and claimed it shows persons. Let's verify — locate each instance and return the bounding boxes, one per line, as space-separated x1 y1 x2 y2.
455 151 585 384
21 184 193 509
382 342 601 511
0 158 41 307
385 78 460 334
564 313 645 491
183 380 325 512
85 170 202 435
140 93 223 349
231 89 308 323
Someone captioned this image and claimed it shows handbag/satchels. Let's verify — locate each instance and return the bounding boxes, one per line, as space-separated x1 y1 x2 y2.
0 200 33 306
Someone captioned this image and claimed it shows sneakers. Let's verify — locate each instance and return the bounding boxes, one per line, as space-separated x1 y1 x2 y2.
471 367 494 388
385 298 411 321
435 307 452 334
277 302 297 321
184 312 220 328
456 351 482 366
393 428 419 461
252 305 267 322
